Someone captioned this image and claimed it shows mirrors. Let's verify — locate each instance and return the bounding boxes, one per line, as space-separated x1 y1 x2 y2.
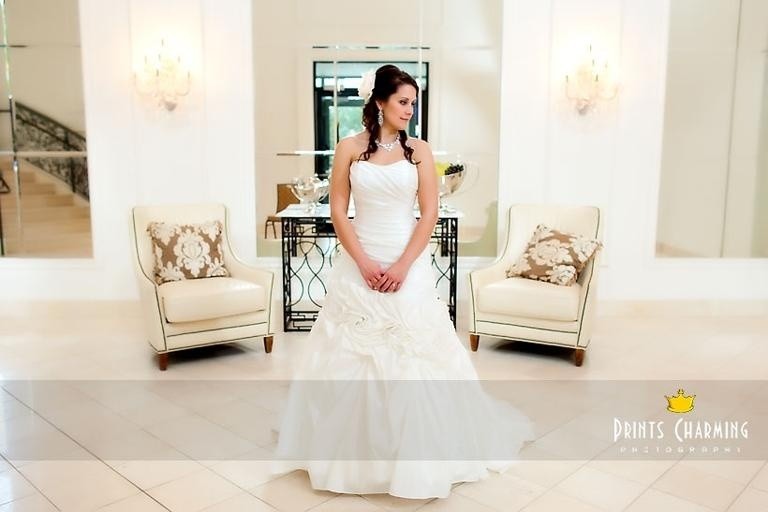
251 0 504 258
655 0 768 259
0 0 94 259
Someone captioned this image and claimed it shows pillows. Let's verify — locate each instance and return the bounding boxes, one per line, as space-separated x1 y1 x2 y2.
146 220 231 286
506 222 603 287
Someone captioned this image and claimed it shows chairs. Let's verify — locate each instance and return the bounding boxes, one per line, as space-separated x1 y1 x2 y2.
265 184 300 239
129 203 279 371
466 203 605 366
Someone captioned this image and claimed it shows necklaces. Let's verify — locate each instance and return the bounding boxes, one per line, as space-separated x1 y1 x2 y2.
375 133 401 152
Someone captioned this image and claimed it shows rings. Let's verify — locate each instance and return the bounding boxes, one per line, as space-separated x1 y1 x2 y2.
392 284 395 287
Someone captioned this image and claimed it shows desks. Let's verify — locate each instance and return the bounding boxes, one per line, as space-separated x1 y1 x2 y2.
275 210 463 332
285 203 456 258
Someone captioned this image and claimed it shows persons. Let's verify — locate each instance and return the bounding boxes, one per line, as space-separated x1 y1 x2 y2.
265 64 535 499
